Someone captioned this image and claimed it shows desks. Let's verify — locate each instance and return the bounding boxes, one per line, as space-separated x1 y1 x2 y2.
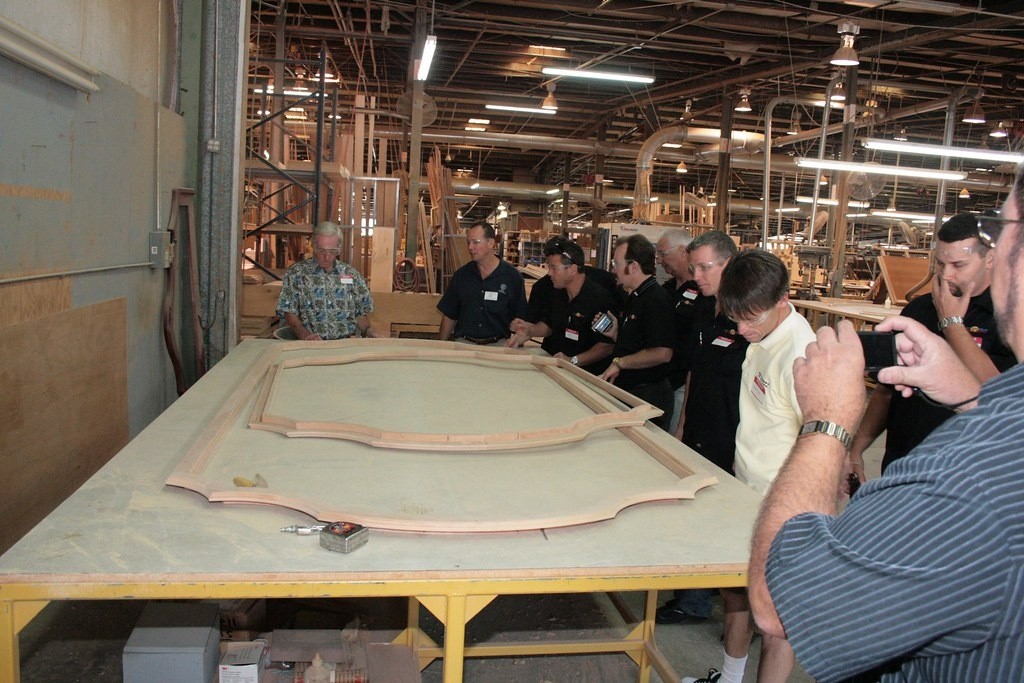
788 296 905 335
0 336 767 683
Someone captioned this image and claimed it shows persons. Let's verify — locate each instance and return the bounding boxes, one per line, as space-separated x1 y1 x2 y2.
666 250 821 683
654 227 700 435
435 221 527 349
275 221 384 341
505 236 613 381
656 231 751 625
746 165 1024 683
841 212 1016 495
591 234 676 432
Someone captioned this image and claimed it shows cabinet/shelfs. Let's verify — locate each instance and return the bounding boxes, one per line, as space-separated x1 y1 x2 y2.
518 241 548 268
503 230 560 267
565 227 601 268
509 210 544 232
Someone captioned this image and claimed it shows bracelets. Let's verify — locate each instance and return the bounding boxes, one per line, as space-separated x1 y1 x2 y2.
364 325 370 335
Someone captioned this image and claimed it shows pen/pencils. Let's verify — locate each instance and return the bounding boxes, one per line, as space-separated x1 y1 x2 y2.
280 523 327 535
568 316 571 324
700 332 702 345
623 317 628 327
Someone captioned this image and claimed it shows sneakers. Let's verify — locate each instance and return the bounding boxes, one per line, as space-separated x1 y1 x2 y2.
656 601 688 625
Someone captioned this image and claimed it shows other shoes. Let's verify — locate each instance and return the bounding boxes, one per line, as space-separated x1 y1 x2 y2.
681 666 721 683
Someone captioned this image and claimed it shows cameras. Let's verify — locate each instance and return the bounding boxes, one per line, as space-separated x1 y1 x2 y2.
591 313 613 333
856 331 898 370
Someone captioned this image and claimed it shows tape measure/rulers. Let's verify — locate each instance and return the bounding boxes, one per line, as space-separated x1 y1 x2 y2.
320 522 370 553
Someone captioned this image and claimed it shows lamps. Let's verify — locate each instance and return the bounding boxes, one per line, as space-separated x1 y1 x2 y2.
830 82 847 101
820 175 829 186
485 103 557 115
894 128 908 142
417 0 438 81
988 121 1007 138
869 208 952 222
886 198 896 211
542 80 559 110
793 155 968 182
539 63 657 84
444 143 452 163
679 99 695 122
786 112 803 135
314 52 335 79
828 20 861 66
676 161 688 173
961 87 987 124
293 69 310 91
327 104 343 120
734 88 754 112
602 176 614 183
795 195 870 209
958 187 971 199
256 108 270 116
859 136 1024 163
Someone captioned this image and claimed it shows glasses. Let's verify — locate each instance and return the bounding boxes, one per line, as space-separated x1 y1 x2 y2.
974 208 1024 247
688 256 728 274
611 258 635 268
465 238 491 247
314 243 338 256
728 305 772 327
552 242 574 264
655 245 679 260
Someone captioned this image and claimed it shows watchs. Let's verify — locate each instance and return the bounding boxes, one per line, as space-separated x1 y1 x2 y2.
612 357 621 369
937 316 964 331
571 356 578 365
797 420 851 449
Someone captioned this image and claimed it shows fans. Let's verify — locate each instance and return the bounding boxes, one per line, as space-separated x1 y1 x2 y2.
803 210 829 238
588 198 609 211
994 162 1019 175
897 222 919 248
567 203 580 217
392 170 409 186
685 191 707 208
396 90 439 128
845 161 887 201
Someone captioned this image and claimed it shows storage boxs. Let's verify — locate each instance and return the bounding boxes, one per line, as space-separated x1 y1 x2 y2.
590 257 597 264
121 602 221 683
576 236 590 248
200 598 267 642
583 249 590 263
218 641 266 683
371 226 398 294
